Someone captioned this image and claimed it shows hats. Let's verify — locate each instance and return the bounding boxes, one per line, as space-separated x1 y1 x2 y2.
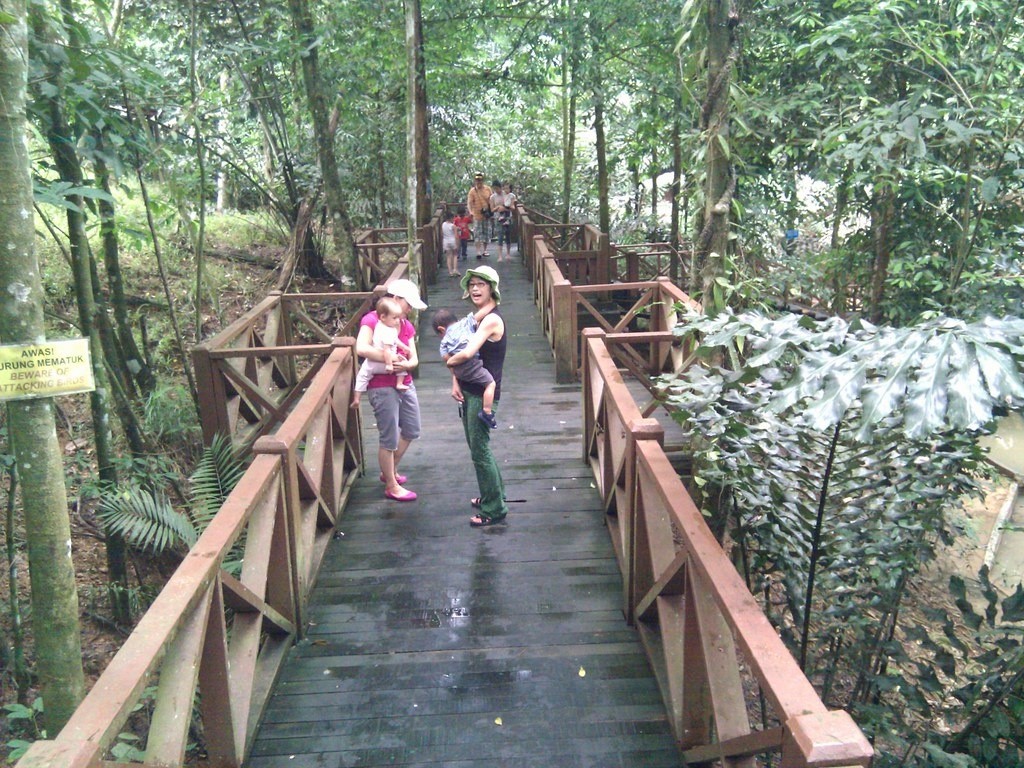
475 174 483 180
387 280 428 310
461 265 501 306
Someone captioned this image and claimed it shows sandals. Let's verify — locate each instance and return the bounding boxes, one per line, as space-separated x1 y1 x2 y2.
477 255 481 258
470 513 505 526
472 497 482 508
483 253 490 256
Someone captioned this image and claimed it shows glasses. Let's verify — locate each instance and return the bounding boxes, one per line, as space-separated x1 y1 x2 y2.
467 282 489 289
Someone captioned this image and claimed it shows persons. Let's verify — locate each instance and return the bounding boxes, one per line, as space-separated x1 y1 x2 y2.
441 211 461 276
497 183 517 226
467 173 494 259
452 206 474 260
348 295 415 408
446 264 511 528
489 179 513 263
432 299 500 430
353 282 429 501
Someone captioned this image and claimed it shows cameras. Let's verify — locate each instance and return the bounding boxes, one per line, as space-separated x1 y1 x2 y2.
458 401 465 417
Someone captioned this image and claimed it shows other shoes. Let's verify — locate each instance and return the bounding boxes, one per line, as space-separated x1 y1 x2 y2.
453 270 461 275
380 474 406 485
448 274 454 277
384 487 417 501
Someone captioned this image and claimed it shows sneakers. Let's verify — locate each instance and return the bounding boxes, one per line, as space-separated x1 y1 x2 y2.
478 409 498 428
